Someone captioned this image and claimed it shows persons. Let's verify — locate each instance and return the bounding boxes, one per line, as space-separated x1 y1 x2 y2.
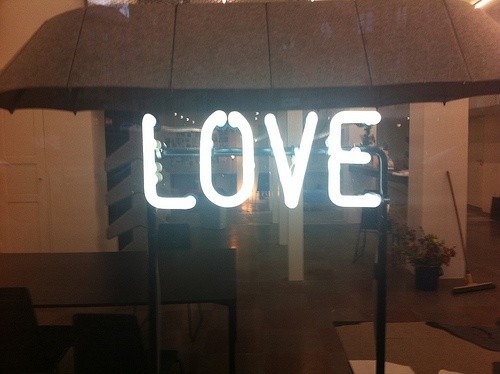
373 142 394 168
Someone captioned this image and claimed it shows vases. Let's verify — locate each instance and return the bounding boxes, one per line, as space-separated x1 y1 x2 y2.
410 268 443 297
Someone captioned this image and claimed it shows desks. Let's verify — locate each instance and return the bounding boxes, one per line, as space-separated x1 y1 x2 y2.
1 249 238 373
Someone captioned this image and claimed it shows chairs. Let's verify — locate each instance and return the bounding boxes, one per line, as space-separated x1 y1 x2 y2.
157 222 204 334
2 286 75 370
349 190 400 265
67 313 151 373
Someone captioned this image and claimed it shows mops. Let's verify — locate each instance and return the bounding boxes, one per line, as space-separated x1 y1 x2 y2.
445 170 497 296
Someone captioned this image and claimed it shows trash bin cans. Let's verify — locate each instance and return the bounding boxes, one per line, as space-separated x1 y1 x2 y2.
490 195 500 220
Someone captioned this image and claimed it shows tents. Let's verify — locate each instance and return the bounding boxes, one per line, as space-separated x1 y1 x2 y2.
1 1 500 374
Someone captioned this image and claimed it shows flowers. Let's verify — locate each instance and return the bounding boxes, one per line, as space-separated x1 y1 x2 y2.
390 224 458 270
